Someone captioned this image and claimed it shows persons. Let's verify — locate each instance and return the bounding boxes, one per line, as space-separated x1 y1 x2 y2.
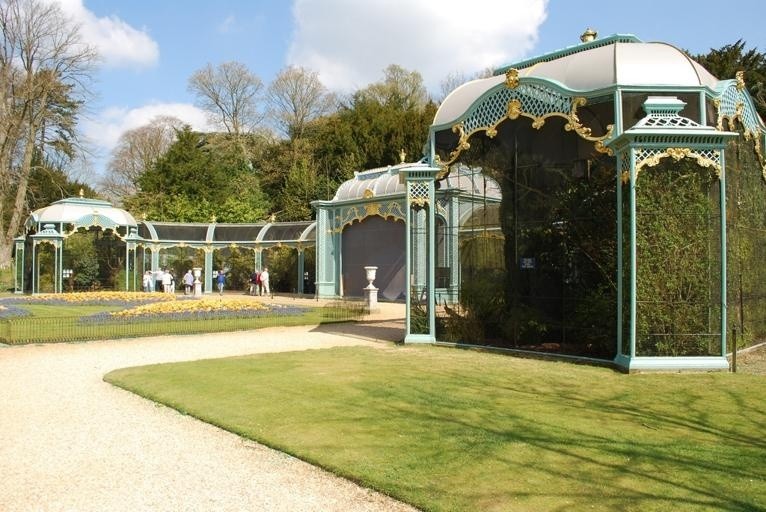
216 270 225 296
249 267 271 297
142 268 173 293
182 270 193 294
